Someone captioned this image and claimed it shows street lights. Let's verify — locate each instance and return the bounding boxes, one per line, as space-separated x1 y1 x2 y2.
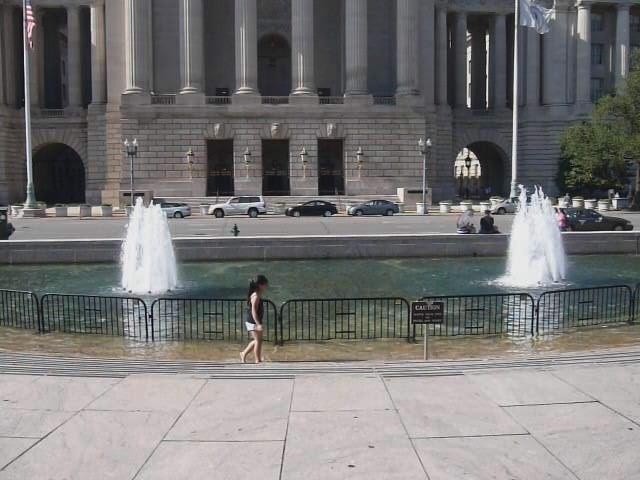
417 137 433 203
122 138 139 206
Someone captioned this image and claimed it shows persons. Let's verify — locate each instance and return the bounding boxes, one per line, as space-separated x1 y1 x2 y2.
238 274 269 363
454 209 476 234
556 207 568 232
477 209 500 235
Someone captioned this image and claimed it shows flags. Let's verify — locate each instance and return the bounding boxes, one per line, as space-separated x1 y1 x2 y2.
518 0 554 35
25 0 40 51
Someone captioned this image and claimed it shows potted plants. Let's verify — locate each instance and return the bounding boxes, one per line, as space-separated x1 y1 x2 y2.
558 195 631 212
394 196 505 215
8 202 136 217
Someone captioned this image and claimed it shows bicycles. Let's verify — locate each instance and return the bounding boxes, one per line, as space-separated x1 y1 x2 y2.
284 199 339 217
346 199 400 217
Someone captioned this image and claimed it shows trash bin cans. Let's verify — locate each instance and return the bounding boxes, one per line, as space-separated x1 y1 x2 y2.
0 208 10 240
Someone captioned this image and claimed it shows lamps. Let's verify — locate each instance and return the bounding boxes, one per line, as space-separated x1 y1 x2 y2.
185 148 195 166
357 146 363 162
244 147 252 163
300 147 308 163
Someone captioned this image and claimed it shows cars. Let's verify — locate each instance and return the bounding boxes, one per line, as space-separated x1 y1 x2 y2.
488 195 533 215
559 206 634 231
158 201 192 218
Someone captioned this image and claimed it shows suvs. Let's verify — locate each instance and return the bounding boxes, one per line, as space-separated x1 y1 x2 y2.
206 194 267 218
